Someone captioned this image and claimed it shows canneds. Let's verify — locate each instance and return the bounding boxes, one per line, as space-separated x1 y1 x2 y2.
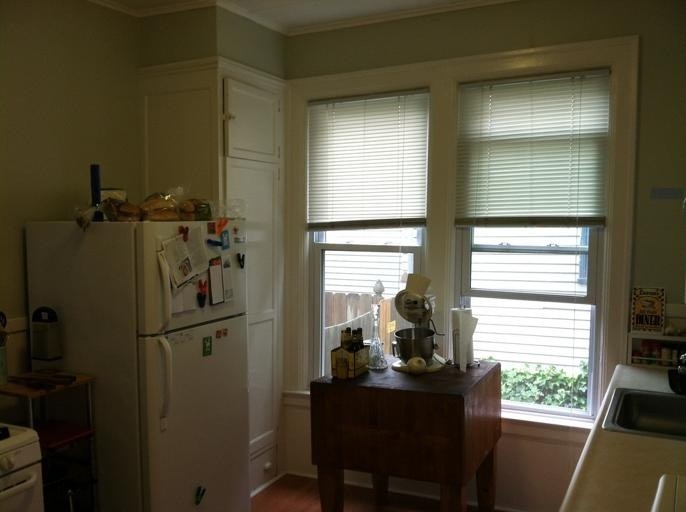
197 205 210 220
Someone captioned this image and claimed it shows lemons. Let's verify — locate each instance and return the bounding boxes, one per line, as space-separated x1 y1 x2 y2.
407 356 426 375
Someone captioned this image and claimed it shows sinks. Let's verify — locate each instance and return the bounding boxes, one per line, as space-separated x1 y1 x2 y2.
600 386 685 441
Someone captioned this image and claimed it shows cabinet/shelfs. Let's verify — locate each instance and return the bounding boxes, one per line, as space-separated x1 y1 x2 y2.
0 372 96 512
146 69 286 500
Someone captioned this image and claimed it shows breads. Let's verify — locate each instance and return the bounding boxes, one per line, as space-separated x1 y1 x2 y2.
118 194 199 221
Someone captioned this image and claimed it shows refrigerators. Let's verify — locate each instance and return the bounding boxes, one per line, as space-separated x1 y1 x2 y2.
20 213 250 511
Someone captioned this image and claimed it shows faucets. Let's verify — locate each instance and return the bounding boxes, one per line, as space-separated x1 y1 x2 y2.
677 353 685 373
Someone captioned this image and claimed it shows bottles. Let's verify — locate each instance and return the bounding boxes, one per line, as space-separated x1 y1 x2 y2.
633 342 677 367
338 327 363 351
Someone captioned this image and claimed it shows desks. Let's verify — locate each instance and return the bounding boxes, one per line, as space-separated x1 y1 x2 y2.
309 358 500 512
